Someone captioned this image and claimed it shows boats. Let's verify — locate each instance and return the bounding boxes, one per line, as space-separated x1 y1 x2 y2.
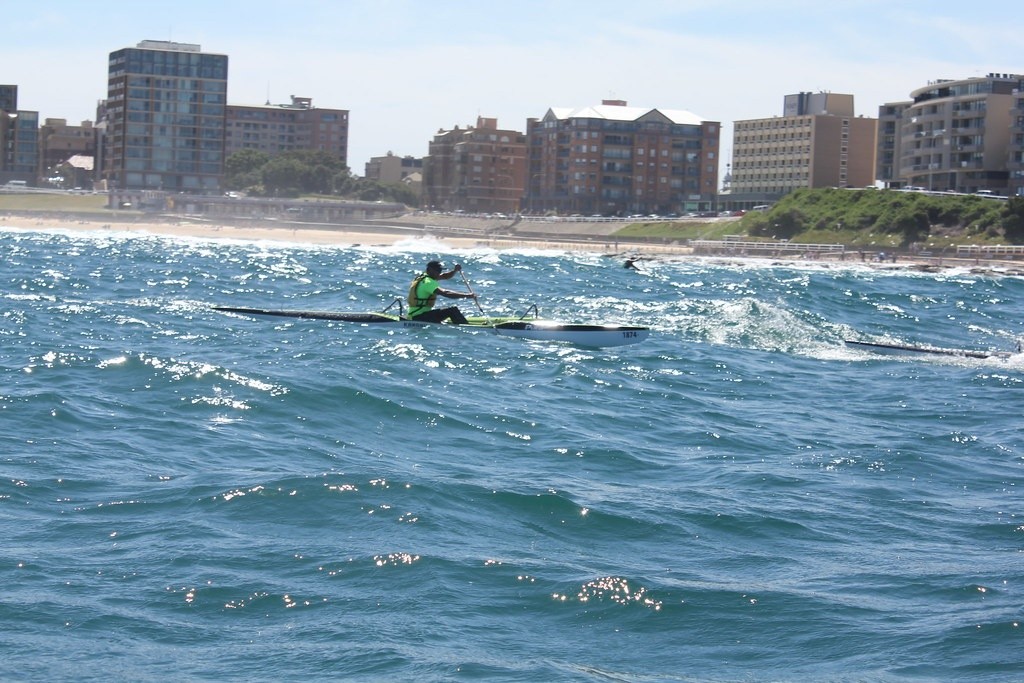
211 307 650 348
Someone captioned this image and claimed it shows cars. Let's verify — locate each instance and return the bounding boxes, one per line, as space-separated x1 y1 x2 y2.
438 204 774 220
890 185 994 198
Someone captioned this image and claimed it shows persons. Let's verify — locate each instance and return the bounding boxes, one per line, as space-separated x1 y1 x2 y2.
404 259 477 330
798 245 915 264
622 256 642 270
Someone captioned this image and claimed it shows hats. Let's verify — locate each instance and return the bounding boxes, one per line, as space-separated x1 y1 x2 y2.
426 261 447 270
